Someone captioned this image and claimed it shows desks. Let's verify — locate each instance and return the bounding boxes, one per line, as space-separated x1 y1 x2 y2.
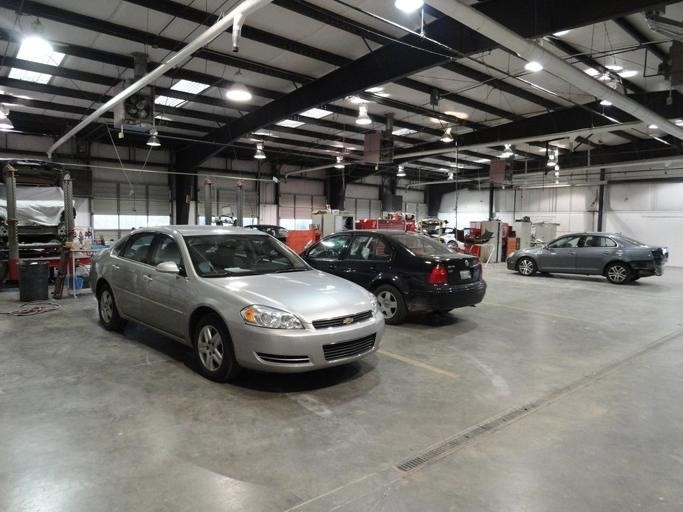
66 249 100 298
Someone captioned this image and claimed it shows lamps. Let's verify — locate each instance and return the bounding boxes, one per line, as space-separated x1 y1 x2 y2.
546 147 558 167
335 156 345 169
146 130 162 146
440 128 454 142
355 104 371 125
254 142 266 159
226 82 252 101
0 107 14 128
396 165 406 177
502 144 513 156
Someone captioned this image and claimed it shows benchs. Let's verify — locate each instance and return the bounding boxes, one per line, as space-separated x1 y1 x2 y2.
131 242 183 269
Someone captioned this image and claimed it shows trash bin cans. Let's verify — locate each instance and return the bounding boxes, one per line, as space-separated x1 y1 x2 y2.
16 261 48 302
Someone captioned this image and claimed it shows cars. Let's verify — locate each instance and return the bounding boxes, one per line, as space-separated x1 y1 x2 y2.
290 229 487 324
243 224 289 243
506 232 669 284
424 227 465 252
86 226 385 382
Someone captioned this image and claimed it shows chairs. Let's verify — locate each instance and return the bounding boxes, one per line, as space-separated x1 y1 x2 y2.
178 245 210 277
212 245 235 270
355 242 372 255
